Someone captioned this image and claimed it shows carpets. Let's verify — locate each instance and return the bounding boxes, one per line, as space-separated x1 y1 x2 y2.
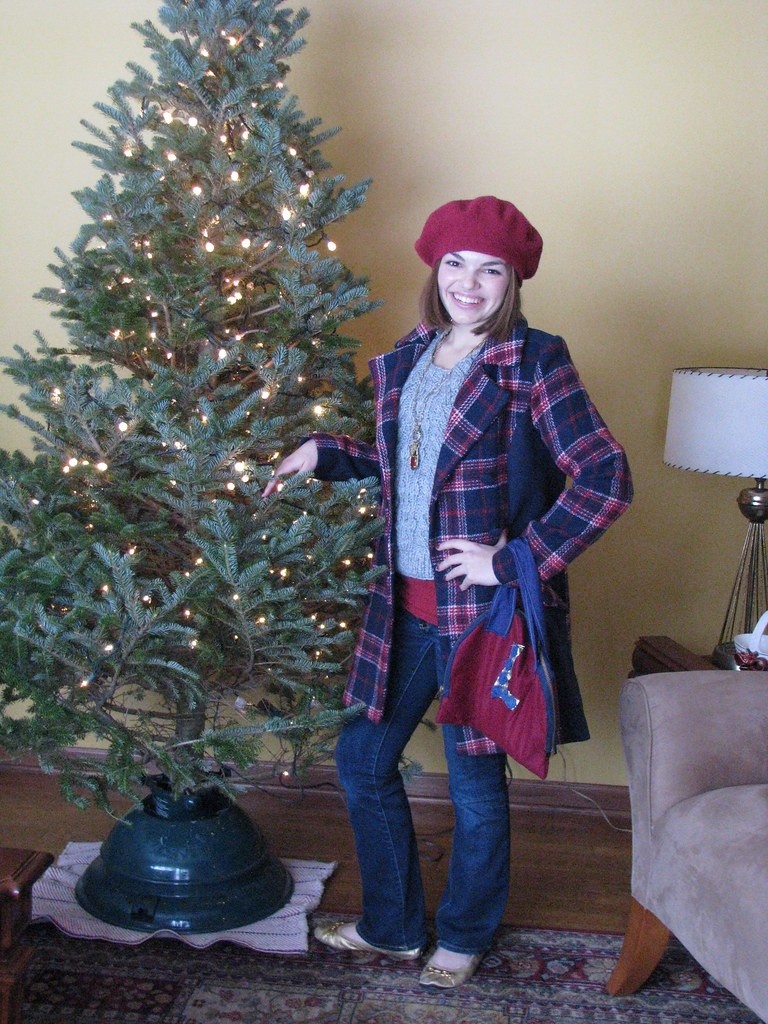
10 908 764 1024
25 833 344 959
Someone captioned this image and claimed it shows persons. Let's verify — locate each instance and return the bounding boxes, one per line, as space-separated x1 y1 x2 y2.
259 196 634 993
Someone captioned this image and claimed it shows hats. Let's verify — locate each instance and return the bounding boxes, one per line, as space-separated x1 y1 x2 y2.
415 196 543 287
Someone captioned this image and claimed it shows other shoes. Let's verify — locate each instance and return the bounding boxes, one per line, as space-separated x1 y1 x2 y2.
314 920 422 961
419 948 484 988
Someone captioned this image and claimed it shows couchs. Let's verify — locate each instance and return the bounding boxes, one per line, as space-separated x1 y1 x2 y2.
600 670 768 1024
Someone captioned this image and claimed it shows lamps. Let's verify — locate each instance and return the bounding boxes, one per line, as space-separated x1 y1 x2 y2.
663 367 768 669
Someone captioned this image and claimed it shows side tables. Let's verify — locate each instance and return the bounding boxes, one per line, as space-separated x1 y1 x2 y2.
627 636 716 678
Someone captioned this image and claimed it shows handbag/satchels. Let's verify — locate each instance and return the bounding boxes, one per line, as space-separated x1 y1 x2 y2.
435 538 558 779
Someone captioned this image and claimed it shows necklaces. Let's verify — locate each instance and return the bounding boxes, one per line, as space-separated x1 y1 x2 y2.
408 331 487 470
